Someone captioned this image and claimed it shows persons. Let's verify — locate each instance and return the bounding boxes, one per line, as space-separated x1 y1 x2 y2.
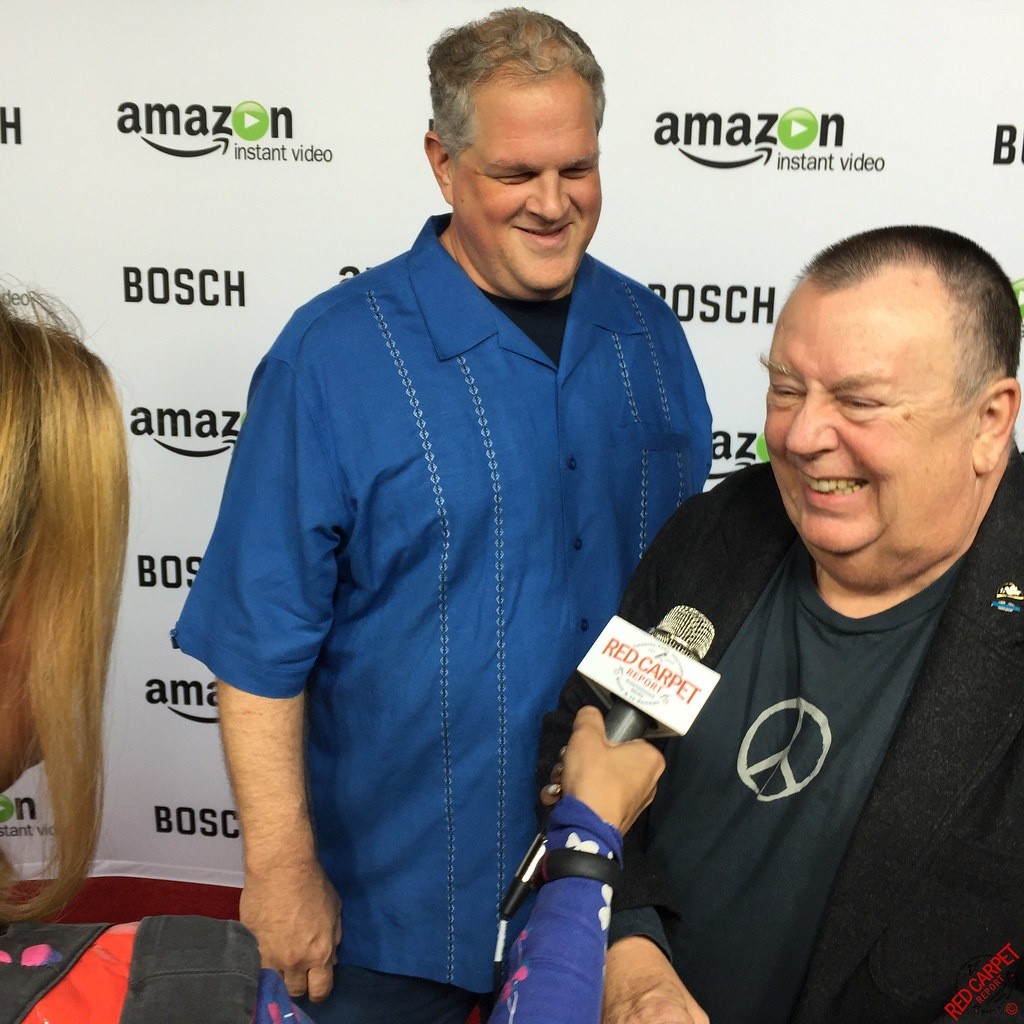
175 9 711 1024
533 226 1024 1024
0 307 666 1024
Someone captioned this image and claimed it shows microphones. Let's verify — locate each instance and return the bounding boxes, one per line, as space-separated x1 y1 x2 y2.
497 604 720 915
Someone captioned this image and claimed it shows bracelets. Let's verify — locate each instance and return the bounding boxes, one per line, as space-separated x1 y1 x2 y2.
545 848 617 888
